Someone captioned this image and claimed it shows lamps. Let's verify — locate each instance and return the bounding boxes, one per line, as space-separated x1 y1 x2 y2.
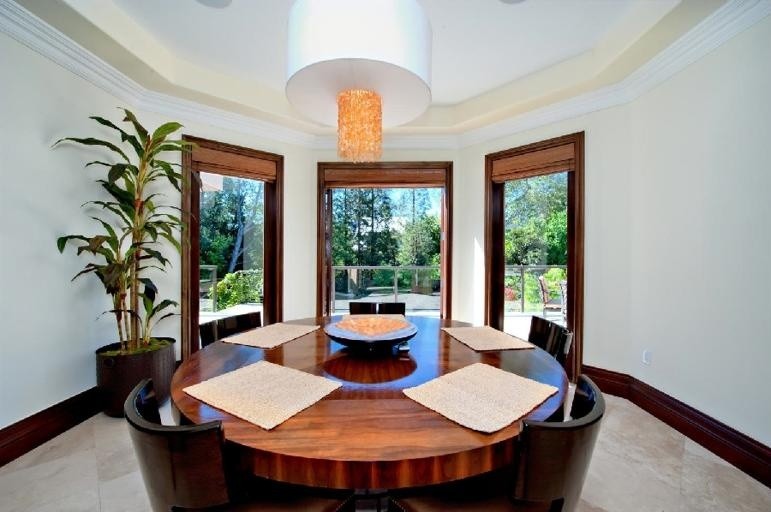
284 2 435 165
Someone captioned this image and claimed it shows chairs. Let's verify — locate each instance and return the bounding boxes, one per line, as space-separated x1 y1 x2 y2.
539 276 563 320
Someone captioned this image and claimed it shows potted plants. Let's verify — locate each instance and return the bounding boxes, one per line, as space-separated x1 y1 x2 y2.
51 105 205 419
